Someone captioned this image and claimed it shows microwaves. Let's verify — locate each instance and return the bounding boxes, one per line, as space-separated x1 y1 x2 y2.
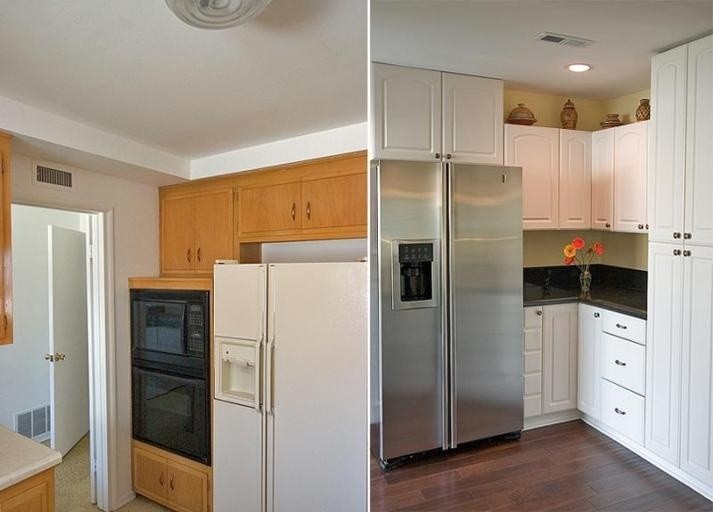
131 288 207 376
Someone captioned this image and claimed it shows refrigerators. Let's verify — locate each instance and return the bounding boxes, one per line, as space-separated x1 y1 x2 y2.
378 157 524 462
213 259 368 512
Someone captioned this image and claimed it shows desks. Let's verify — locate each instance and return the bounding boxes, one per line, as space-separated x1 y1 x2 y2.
0 464 62 512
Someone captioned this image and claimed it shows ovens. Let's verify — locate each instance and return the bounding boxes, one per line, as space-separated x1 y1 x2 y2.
129 367 210 466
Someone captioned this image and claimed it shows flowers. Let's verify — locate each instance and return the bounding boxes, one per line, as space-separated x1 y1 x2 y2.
561 236 608 292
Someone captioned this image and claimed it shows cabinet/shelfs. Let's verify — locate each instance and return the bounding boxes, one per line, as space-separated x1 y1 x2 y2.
239 149 367 244
504 123 560 231
560 128 592 230
158 172 262 278
644 34 713 502
599 309 647 447
130 439 213 512
523 302 577 419
370 62 505 167
592 127 614 232
614 120 647 233
577 302 600 420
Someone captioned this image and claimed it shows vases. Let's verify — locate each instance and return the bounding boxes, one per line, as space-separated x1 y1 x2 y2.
578 271 593 302
